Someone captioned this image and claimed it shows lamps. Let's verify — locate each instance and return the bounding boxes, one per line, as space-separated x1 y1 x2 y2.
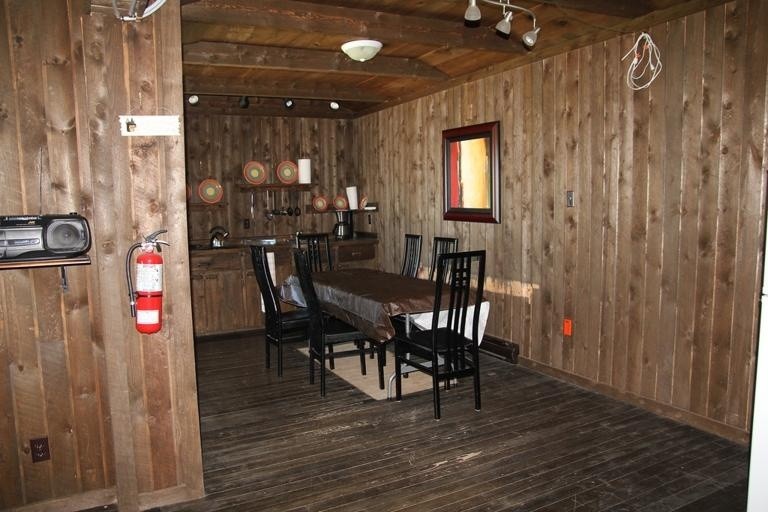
340 39 383 63
464 1 541 48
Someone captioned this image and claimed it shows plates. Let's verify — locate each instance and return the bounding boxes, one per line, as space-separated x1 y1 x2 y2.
333 196 348 211
313 196 329 213
198 179 224 204
276 160 298 185
243 162 266 184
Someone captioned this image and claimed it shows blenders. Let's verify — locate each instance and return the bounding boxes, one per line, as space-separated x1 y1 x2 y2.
333 210 354 241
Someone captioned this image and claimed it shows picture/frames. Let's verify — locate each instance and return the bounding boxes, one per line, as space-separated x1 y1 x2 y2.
439 120 503 226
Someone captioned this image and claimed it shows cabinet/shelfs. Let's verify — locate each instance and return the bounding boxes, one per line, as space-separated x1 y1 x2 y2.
188 238 379 339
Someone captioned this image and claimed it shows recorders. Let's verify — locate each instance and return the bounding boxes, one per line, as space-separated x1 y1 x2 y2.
0 212 92 263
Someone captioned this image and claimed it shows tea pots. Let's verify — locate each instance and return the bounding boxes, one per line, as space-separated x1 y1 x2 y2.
209 226 229 248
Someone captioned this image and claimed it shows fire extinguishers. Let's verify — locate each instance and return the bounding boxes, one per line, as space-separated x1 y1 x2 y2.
125 228 171 335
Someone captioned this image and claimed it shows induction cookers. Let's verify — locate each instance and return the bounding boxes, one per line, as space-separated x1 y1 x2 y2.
189 242 244 250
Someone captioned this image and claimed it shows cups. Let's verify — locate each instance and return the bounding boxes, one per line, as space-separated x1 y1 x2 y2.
345 186 359 209
